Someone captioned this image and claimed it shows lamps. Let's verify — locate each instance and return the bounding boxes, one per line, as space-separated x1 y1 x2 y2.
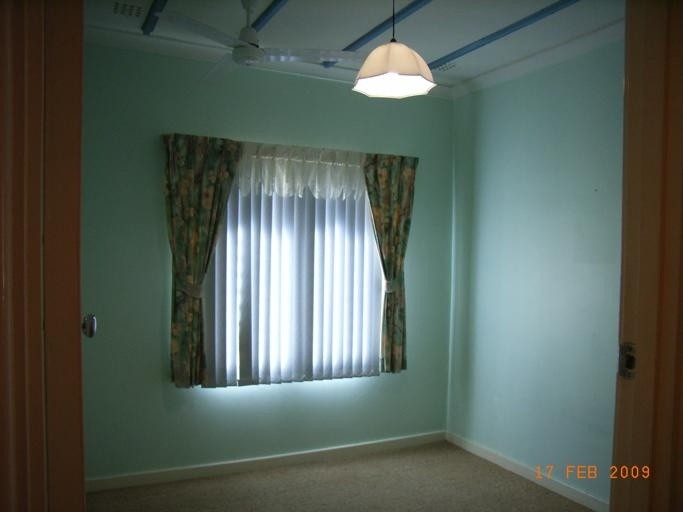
350 0 439 100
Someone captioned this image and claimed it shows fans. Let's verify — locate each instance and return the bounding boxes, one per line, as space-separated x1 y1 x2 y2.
153 0 368 70
